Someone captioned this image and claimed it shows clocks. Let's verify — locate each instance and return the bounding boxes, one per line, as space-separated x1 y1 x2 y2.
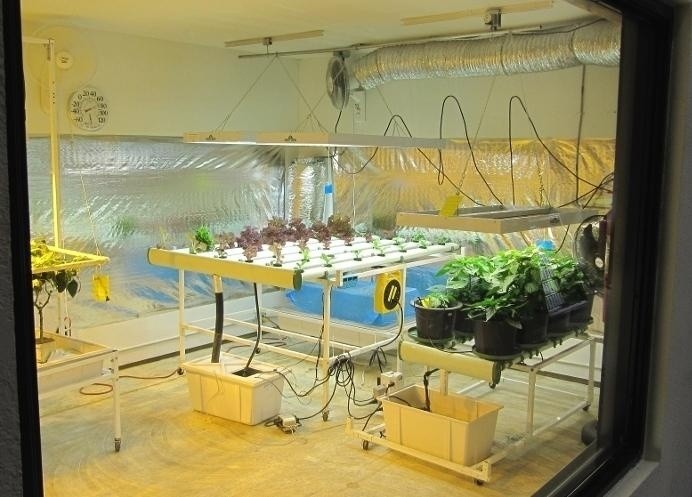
67 86 108 131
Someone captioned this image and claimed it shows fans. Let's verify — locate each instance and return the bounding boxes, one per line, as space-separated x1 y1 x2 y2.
325 54 365 123
573 214 607 445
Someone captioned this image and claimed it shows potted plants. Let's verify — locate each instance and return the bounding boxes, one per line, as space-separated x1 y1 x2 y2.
409 244 595 360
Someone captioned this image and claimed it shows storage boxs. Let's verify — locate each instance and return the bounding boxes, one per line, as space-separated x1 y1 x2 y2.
183 351 288 426
377 384 504 465
